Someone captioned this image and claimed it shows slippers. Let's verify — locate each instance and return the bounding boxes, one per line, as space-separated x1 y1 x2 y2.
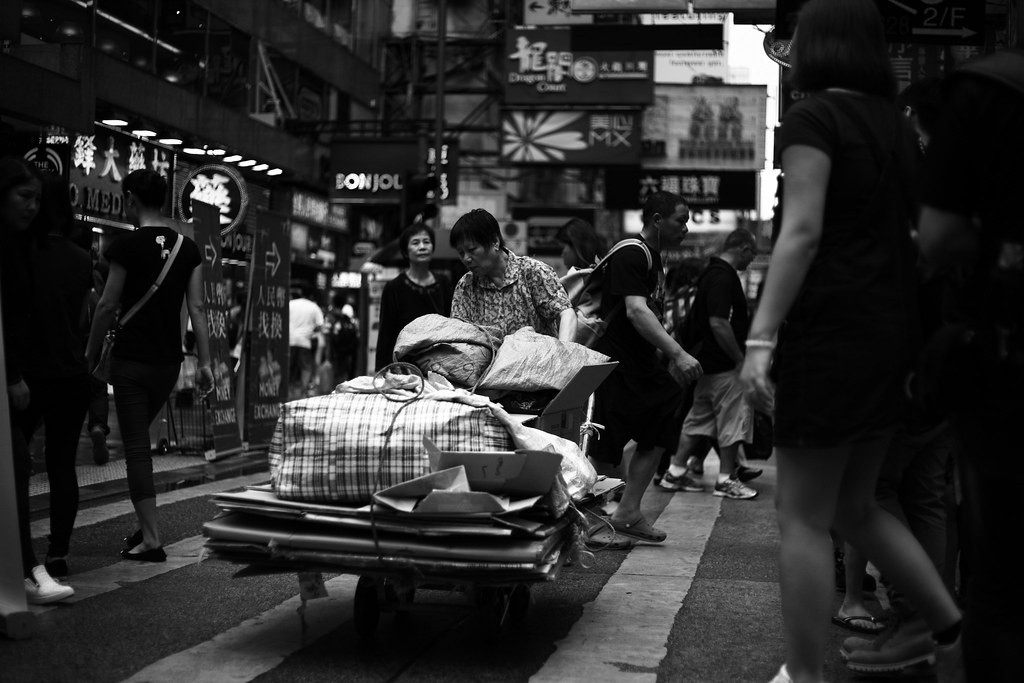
832 614 886 634
583 525 630 550
606 516 667 543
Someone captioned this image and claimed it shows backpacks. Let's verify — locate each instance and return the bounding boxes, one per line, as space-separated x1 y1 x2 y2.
673 264 735 348
559 239 651 348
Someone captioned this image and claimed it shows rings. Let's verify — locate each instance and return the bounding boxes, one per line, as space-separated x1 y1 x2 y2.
746 389 755 394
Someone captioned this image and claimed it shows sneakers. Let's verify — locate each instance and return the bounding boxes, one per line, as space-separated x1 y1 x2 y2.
660 469 705 493
712 478 758 500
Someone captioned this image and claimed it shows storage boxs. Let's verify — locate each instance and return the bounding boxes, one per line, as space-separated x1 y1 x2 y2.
507 361 620 446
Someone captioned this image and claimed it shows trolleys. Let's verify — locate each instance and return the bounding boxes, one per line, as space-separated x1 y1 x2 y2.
153 349 215 459
349 389 598 638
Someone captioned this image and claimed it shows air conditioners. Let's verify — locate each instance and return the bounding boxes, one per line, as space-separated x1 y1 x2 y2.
499 222 527 240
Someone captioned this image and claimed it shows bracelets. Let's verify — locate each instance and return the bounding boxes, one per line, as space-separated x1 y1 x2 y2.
745 339 775 350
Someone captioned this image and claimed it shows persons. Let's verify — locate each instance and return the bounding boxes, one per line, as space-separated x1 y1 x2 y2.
289 288 361 398
736 0 1024 683
374 223 456 380
85 170 215 564
450 209 579 343
658 233 763 502
571 188 704 541
27 173 112 464
0 155 90 602
555 219 629 502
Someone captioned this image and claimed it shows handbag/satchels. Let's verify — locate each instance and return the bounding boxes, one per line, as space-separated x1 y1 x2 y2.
88 329 119 385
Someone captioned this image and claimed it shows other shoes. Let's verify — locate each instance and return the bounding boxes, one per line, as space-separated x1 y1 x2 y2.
654 477 660 484
839 622 961 671
729 465 763 483
125 529 144 547
686 464 703 477
24 565 74 604
863 573 877 594
120 546 166 564
92 425 110 466
45 559 68 582
